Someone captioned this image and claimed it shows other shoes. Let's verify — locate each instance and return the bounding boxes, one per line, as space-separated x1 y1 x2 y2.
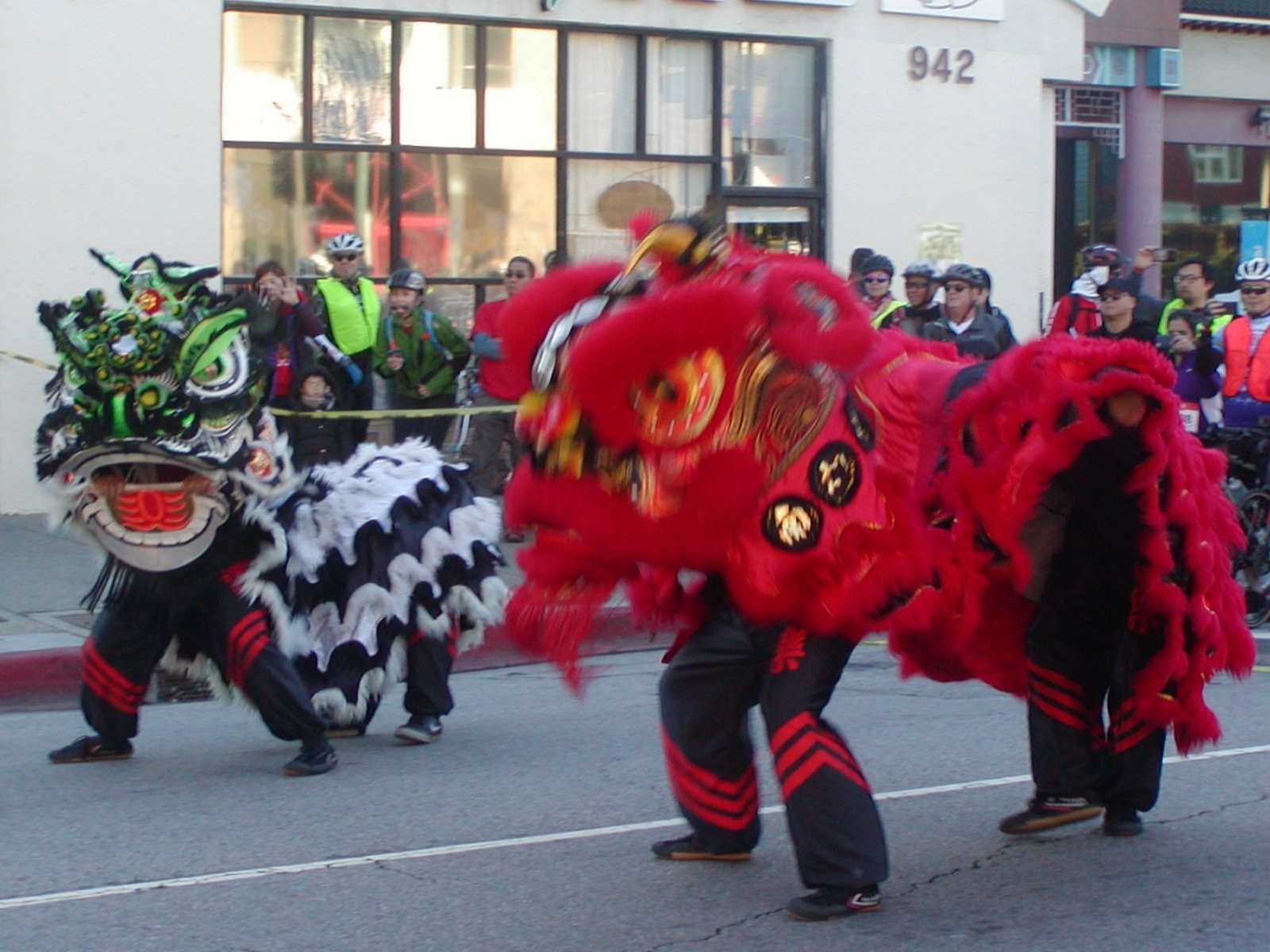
323 728 360 739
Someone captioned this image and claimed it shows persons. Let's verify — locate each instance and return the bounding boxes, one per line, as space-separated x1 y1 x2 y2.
1193 256 1270 630
231 219 1240 543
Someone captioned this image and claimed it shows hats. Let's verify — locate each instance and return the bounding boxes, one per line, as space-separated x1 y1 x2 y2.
1096 278 1135 297
975 267 991 289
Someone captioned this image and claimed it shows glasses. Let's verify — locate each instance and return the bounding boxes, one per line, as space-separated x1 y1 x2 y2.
504 271 527 279
1098 294 1122 302
1172 274 1205 283
905 281 930 289
944 284 966 292
331 252 357 262
863 275 890 284
1240 286 1270 295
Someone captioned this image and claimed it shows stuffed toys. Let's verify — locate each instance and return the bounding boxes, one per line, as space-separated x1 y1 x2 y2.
450 209 1261 924
28 241 518 779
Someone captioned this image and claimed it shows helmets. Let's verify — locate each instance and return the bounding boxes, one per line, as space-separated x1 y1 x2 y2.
1081 242 1133 271
942 262 987 288
324 231 364 254
858 255 894 276
1234 258 1270 282
386 268 428 292
902 261 943 282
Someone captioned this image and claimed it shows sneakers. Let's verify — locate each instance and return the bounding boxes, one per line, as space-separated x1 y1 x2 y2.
284 746 339 777
785 882 884 922
651 835 753 862
395 714 444 743
998 794 1144 837
48 735 135 763
